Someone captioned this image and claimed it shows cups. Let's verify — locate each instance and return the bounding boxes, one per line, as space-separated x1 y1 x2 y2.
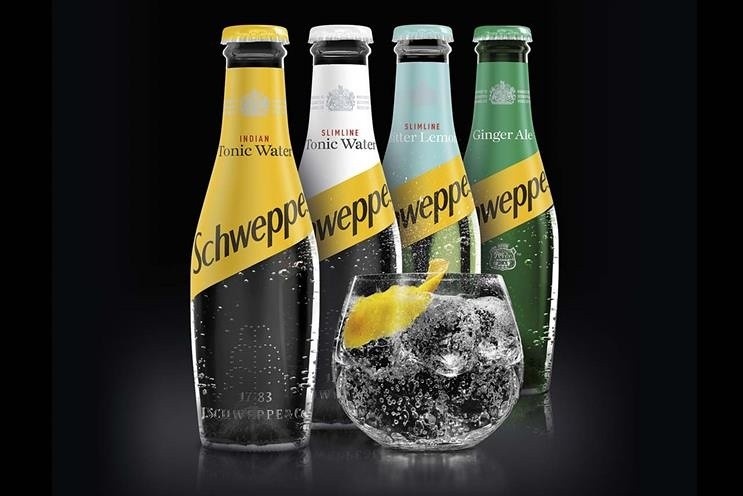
330 273 524 453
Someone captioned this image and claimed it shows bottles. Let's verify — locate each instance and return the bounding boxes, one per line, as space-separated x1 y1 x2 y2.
297 22 404 432
189 25 320 453
463 25 560 396
381 24 482 276
